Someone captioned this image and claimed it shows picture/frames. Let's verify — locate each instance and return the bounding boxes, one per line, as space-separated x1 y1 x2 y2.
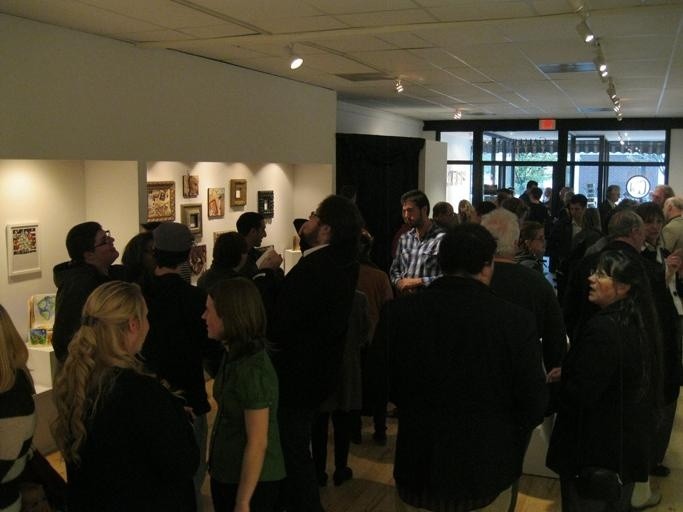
147 181 176 222
230 178 247 206
183 175 198 197
180 203 202 237
208 187 225 217
258 190 275 218
7 223 41 276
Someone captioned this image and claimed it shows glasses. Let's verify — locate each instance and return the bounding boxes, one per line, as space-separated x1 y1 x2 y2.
310 212 319 220
532 234 544 241
92 230 111 248
590 268 615 278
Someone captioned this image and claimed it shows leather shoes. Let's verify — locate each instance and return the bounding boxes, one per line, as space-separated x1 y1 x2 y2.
650 462 670 477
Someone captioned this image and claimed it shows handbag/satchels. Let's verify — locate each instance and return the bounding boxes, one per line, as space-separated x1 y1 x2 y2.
16 442 69 512
573 469 624 501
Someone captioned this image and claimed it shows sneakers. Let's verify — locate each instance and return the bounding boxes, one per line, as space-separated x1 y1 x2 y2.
316 473 328 487
333 467 352 486
375 436 387 446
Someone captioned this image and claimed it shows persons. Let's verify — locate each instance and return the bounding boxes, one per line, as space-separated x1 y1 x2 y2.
52 181 681 510
0 303 39 512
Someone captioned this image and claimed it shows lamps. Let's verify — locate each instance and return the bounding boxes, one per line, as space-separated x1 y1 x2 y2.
594 55 623 121
575 23 596 43
285 42 304 69
391 80 404 92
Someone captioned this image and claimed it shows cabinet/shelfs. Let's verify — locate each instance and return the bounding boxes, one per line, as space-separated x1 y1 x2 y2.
22 341 58 397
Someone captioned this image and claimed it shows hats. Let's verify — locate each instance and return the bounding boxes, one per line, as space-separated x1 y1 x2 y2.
152 223 195 253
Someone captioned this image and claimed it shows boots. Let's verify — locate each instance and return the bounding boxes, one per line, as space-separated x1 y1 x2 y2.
631 476 662 511
